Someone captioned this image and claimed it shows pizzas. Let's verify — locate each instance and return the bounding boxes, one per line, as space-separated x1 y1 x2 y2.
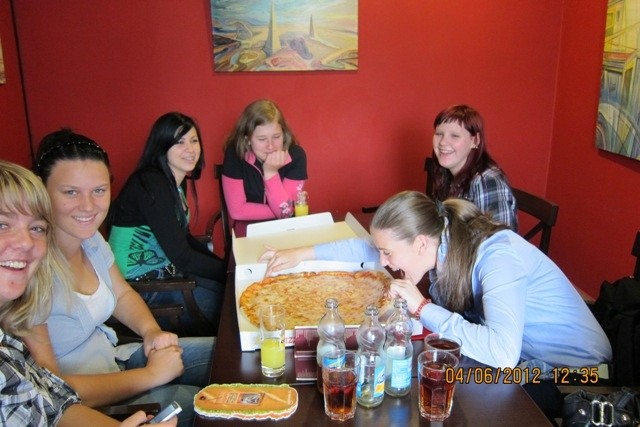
239 270 391 329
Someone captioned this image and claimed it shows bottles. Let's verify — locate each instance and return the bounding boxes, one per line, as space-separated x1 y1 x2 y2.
317 300 346 394
354 307 386 407
385 301 413 398
294 190 309 217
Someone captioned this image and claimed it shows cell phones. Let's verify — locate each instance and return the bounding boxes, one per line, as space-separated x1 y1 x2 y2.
138 399 183 426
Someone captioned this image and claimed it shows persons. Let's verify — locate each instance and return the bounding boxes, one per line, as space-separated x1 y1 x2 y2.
0 160 179 427
221 98 308 222
24 127 214 425
258 190 612 388
104 111 225 338
423 103 519 230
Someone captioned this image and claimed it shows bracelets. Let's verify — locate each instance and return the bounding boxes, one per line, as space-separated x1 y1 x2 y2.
414 299 432 317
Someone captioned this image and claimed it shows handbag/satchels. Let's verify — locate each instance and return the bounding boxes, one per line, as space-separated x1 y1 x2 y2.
564 389 640 427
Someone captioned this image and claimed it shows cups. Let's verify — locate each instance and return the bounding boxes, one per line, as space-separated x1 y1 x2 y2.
321 350 361 421
424 333 463 359
418 351 459 421
259 304 287 377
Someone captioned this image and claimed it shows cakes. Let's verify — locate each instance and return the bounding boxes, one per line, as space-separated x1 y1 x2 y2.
195 383 299 421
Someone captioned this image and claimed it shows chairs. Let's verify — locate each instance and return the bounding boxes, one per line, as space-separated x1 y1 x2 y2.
557 236 639 426
107 202 211 332
508 184 559 254
213 164 232 253
90 300 188 423
